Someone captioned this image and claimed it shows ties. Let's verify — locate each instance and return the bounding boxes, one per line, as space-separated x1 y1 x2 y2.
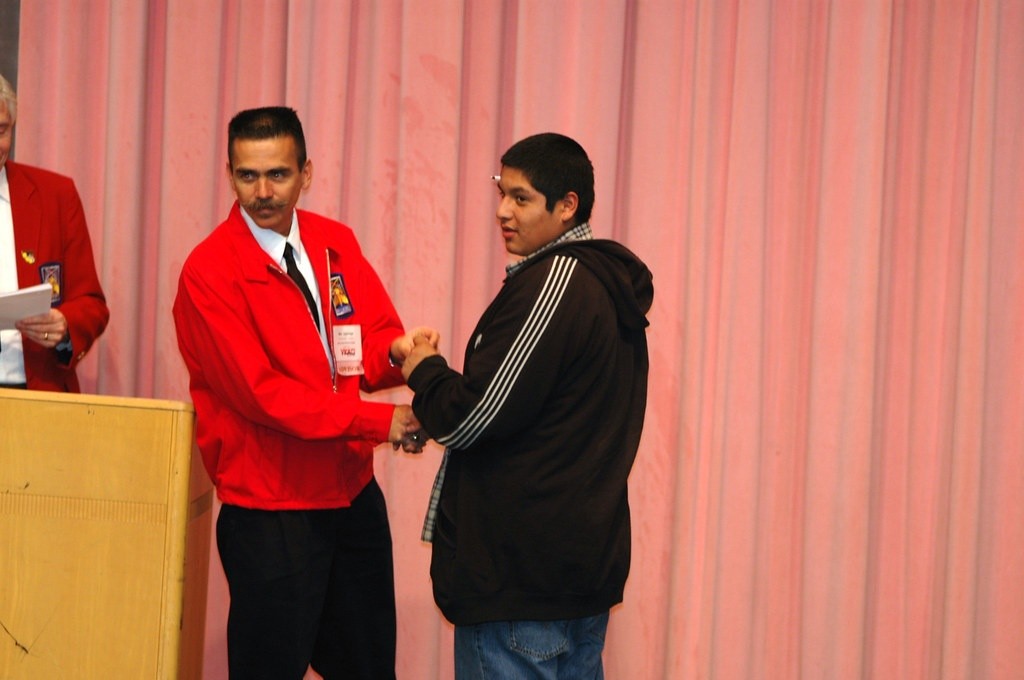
282 244 321 334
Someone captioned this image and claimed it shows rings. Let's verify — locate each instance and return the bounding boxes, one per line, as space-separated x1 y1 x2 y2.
44 332 48 340
408 432 419 441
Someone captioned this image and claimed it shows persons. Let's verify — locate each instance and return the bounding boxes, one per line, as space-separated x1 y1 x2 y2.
172 106 440 680
0 74 111 394
402 132 654 680
